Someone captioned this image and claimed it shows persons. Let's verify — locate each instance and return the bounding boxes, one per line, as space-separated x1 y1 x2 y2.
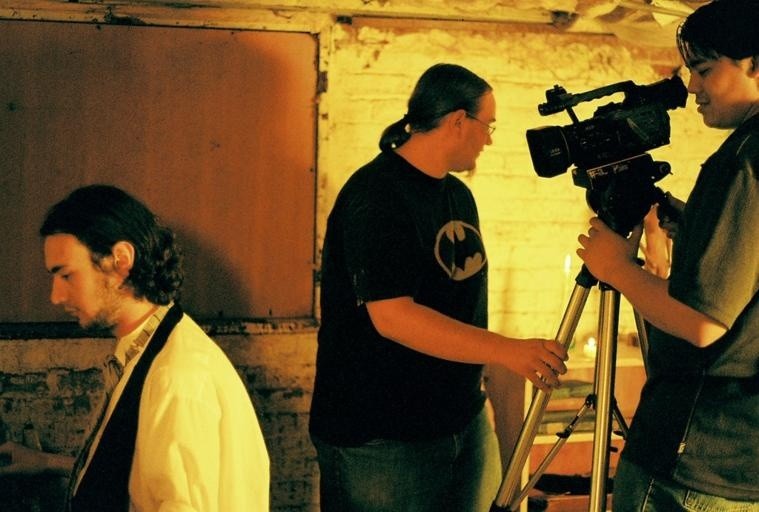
575 0 759 510
636 204 674 280
307 61 571 511
1 183 273 511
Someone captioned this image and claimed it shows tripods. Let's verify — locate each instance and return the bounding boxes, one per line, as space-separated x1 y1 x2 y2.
489 268 660 512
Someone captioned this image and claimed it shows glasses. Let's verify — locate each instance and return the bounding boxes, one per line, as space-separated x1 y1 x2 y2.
464 112 496 135
